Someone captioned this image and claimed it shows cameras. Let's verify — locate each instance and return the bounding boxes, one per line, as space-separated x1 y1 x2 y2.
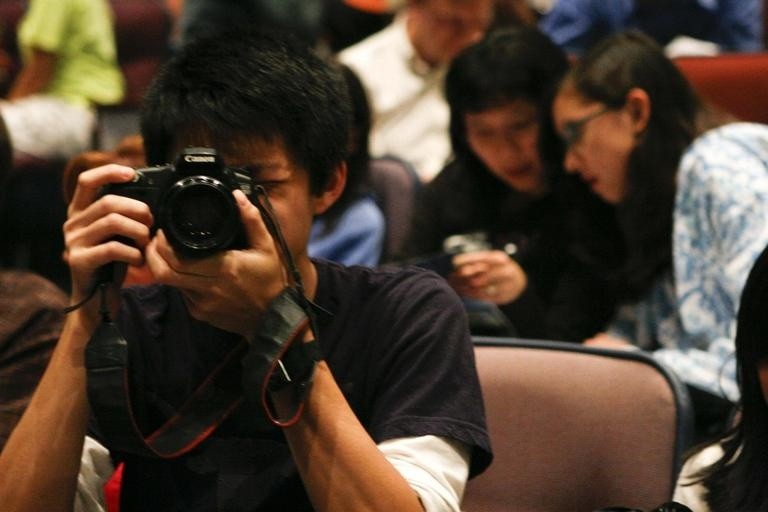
97 147 262 262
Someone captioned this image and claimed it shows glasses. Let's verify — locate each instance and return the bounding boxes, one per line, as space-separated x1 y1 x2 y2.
560 108 605 145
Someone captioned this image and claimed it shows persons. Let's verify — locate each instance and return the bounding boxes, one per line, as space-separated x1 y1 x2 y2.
1 0 768 512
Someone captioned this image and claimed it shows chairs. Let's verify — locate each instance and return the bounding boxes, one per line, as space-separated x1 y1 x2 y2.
673 53 768 124
462 333 692 512
371 154 425 269
